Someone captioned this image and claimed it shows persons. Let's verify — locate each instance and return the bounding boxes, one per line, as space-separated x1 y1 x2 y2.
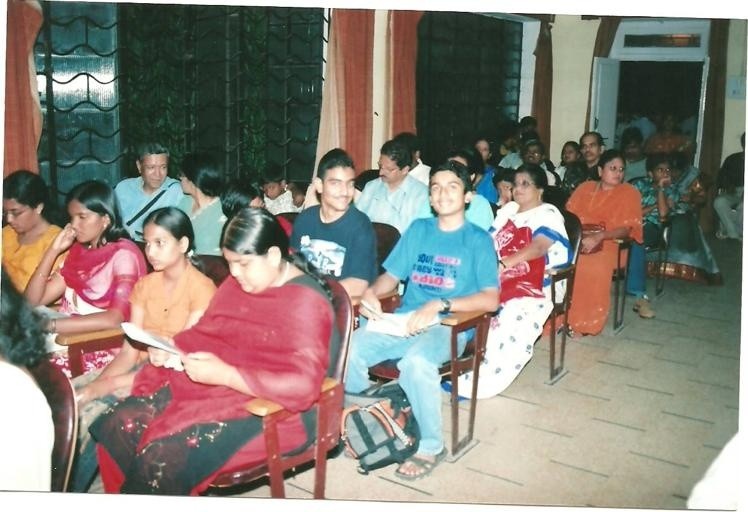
115 141 306 255
713 134 744 239
70 207 215 494
354 116 572 399
543 133 724 337
342 161 500 480
2 169 146 376
89 206 340 495
292 149 378 298
1 270 55 492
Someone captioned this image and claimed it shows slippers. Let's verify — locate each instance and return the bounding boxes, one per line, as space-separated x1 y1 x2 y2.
394 446 447 479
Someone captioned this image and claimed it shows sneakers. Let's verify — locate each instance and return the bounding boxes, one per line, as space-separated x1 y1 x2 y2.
632 302 655 319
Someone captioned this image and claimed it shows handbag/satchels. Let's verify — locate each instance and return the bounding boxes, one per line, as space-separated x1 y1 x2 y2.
343 381 421 474
579 222 604 254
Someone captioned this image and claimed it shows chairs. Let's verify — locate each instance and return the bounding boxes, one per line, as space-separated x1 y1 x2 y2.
0 175 718 501
